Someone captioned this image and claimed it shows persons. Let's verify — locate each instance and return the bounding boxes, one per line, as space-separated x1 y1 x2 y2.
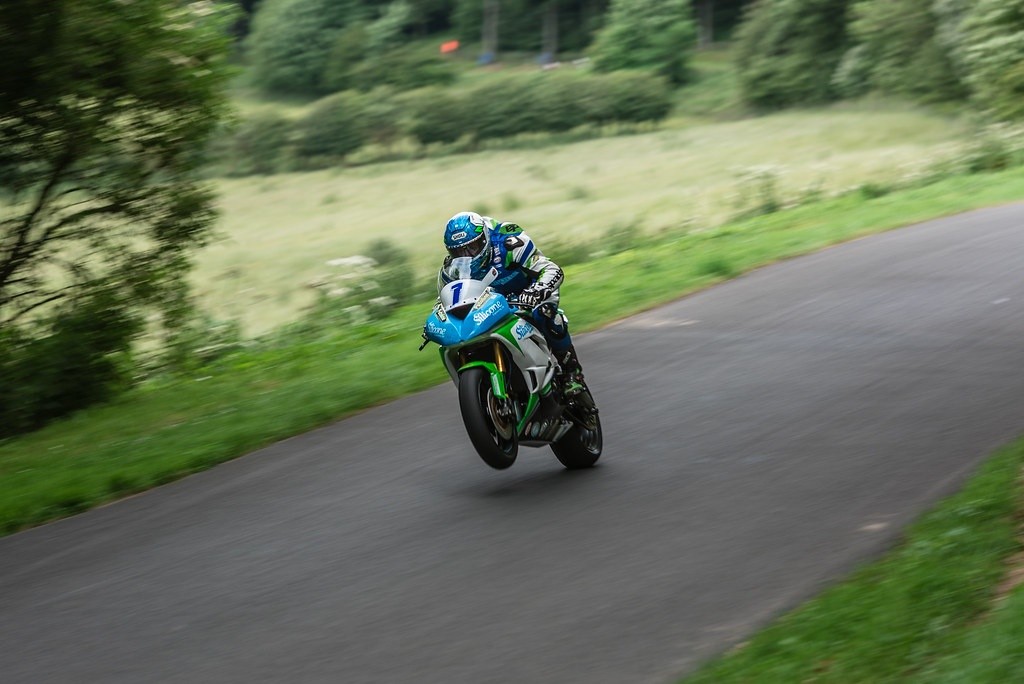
437 211 586 399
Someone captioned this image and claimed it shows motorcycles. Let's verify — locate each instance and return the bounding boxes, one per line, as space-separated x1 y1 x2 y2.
417 251 602 470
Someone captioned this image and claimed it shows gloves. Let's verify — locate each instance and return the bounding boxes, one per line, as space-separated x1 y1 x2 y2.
518 288 540 311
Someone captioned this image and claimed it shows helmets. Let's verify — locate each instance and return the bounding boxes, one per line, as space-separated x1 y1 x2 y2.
444 212 492 281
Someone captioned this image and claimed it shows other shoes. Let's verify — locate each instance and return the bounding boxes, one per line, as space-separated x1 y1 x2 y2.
564 364 586 395
